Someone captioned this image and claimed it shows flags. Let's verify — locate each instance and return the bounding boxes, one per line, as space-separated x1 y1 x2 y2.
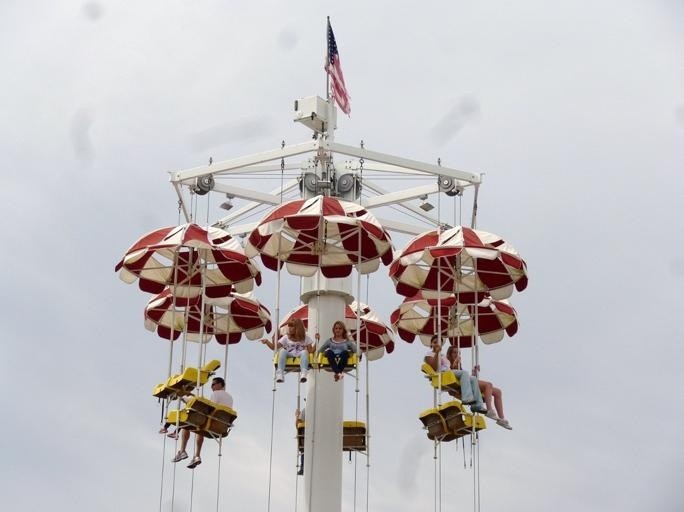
326 21 351 114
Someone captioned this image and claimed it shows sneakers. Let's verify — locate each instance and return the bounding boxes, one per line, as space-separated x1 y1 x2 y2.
186 455 201 469
297 469 303 475
158 427 178 440
275 368 284 383
298 369 308 383
495 417 513 430
469 404 487 414
333 372 343 382
171 450 188 462
461 397 476 405
484 408 500 420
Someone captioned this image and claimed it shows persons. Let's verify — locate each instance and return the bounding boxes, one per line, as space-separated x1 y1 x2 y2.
319 321 357 382
424 335 488 412
295 397 305 475
447 346 512 430
171 378 233 468
260 318 320 382
159 423 181 439
